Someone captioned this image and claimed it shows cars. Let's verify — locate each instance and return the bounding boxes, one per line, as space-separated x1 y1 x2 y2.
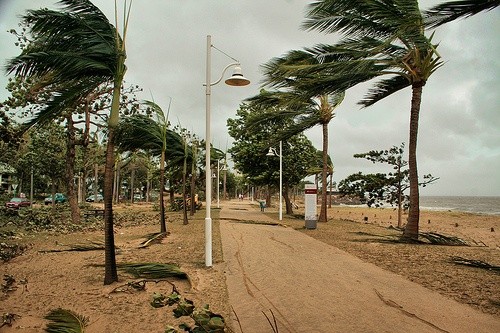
113 190 147 202
86 194 104 202
5 197 31 209
204 35 250 269
44 192 67 205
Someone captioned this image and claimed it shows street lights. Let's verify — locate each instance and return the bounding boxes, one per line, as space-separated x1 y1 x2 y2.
217 156 227 209
267 140 283 222
